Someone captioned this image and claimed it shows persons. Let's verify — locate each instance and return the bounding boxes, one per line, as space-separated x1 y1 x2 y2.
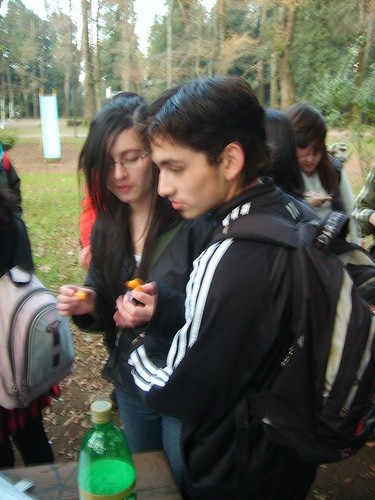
112 75 322 500
55 91 219 495
0 141 62 472
259 103 375 259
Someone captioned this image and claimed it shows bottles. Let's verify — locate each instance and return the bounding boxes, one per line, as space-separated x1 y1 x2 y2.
76 400 138 500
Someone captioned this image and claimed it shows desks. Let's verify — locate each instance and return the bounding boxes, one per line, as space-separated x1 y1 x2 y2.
0 450 182 500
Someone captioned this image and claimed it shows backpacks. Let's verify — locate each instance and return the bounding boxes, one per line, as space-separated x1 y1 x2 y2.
206 210 375 464
0 266 75 410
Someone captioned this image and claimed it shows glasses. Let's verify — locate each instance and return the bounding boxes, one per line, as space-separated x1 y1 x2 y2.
106 152 152 171
